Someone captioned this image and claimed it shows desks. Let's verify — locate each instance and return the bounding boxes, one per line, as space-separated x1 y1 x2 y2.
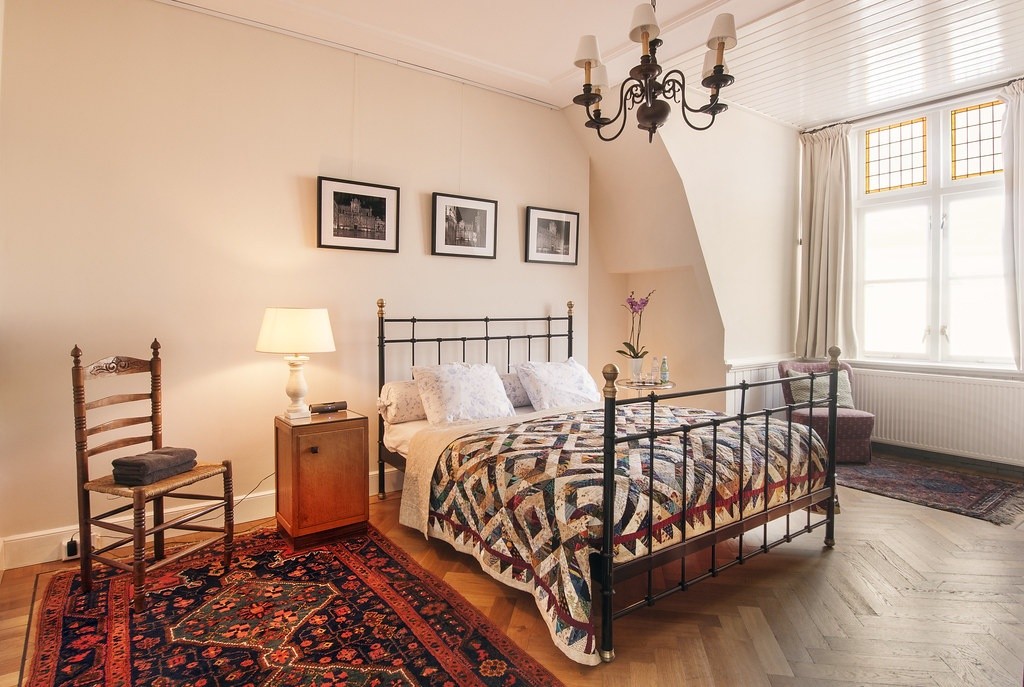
617 378 676 398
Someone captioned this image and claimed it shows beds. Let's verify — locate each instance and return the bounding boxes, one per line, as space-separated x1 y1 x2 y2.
377 298 841 666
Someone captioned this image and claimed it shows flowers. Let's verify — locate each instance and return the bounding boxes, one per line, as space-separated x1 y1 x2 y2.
616 290 657 358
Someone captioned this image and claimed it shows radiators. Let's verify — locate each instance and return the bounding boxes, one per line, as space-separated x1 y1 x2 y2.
852 367 1024 468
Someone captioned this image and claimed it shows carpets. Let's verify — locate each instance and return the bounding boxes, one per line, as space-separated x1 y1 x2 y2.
27 522 570 687
834 453 1024 526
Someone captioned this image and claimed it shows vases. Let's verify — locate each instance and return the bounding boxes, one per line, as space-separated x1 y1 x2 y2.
626 359 643 380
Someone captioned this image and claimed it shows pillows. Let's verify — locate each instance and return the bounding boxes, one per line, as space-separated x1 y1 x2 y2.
411 363 516 426
787 368 855 410
377 374 532 424
509 357 603 411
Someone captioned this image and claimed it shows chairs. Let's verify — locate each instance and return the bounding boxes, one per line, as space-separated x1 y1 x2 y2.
779 361 875 463
70 337 234 613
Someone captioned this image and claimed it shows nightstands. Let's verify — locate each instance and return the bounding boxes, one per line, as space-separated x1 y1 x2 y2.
274 408 370 552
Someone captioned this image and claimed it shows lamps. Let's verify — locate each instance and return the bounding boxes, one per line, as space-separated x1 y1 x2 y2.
255 308 336 421
573 0 737 143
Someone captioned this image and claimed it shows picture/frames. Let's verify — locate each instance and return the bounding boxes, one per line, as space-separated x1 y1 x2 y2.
432 192 498 258
525 206 580 265
317 176 400 253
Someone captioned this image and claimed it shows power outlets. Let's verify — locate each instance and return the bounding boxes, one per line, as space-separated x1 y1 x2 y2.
61 535 98 561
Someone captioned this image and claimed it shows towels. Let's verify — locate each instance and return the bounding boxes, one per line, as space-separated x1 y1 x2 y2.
113 460 197 486
111 447 197 474
